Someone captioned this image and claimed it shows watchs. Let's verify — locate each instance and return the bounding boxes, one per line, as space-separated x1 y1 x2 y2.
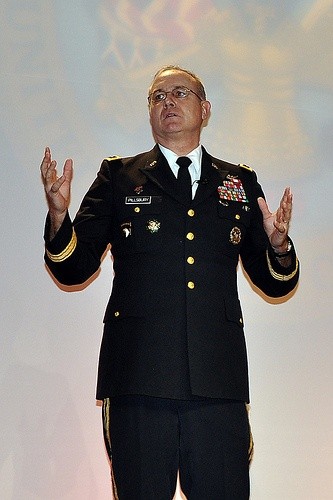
273 238 294 258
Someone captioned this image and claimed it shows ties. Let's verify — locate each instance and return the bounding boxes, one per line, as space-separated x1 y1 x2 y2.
175 156 192 203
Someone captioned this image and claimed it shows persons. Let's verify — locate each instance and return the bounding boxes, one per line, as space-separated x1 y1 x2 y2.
37 63 301 500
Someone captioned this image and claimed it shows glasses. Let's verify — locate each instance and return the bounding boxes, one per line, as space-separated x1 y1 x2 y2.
148 86 202 104
